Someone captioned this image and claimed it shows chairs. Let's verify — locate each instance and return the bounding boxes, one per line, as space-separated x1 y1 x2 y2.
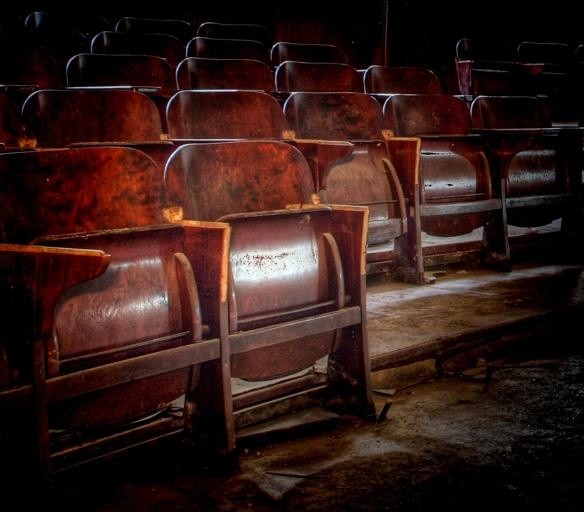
361 65 441 95
1 148 237 467
383 95 507 277
279 66 360 95
174 61 275 92
65 55 176 92
166 141 376 449
23 90 174 181
456 40 516 94
288 95 432 283
161 89 349 205
87 31 182 62
119 18 196 52
269 43 353 70
185 38 268 62
196 21 261 44
468 94 579 269
515 44 572 100
